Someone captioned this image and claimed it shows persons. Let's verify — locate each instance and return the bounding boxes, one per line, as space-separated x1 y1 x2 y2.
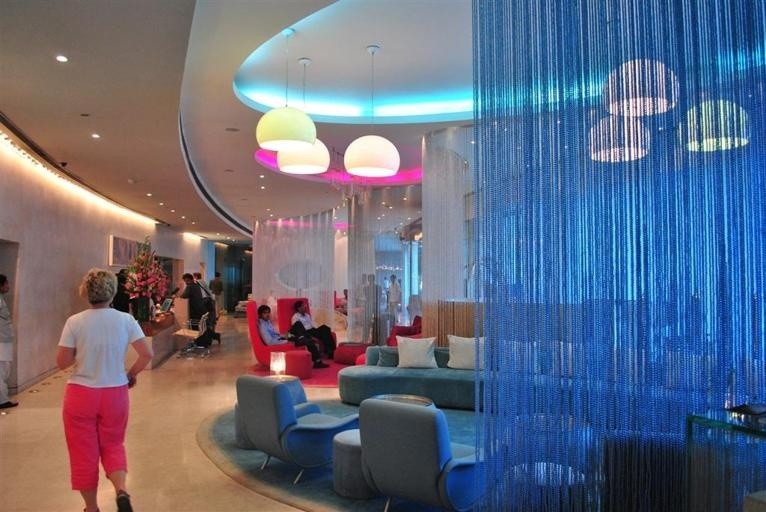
109 268 162 326
179 272 208 349
55 266 155 511
0 274 19 409
258 305 330 369
209 271 223 322
193 272 220 346
340 272 402 346
290 301 337 359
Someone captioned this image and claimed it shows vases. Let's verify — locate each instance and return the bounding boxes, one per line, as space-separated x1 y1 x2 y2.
129 298 149 323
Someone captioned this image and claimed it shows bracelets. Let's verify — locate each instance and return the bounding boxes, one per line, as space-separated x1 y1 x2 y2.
127 374 136 381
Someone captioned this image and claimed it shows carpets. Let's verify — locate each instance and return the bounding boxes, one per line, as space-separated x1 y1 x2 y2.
190 394 606 512
242 349 359 389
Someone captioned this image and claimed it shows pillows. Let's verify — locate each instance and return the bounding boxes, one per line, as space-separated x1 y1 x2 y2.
491 340 762 393
446 335 490 371
396 336 438 370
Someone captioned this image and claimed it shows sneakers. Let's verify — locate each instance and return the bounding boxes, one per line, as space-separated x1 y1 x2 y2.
216 333 220 344
116 490 133 512
296 337 312 346
0 401 18 408
314 361 329 368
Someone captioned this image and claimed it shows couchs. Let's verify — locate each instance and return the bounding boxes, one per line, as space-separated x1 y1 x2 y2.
335 340 766 413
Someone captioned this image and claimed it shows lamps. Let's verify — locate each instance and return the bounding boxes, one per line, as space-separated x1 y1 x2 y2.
600 57 680 118
677 98 756 154
343 44 402 179
277 54 330 175
269 351 289 381
584 115 653 164
255 27 318 153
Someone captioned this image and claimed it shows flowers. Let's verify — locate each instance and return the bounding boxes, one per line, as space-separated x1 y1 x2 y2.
119 231 173 302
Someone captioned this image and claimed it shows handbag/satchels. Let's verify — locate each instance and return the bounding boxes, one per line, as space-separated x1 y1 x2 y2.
203 297 212 312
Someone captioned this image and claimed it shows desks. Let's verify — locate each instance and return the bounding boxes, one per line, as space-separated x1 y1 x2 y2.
687 409 766 511
234 301 248 318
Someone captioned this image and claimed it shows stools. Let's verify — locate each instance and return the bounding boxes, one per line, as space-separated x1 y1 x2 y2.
268 351 312 377
505 462 586 511
331 428 374 500
332 342 367 365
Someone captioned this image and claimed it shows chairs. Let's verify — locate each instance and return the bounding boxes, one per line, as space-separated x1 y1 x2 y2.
234 376 359 486
172 312 212 359
359 397 509 512
245 299 313 375
388 315 422 346
600 428 685 512
275 296 335 353
232 374 308 450
